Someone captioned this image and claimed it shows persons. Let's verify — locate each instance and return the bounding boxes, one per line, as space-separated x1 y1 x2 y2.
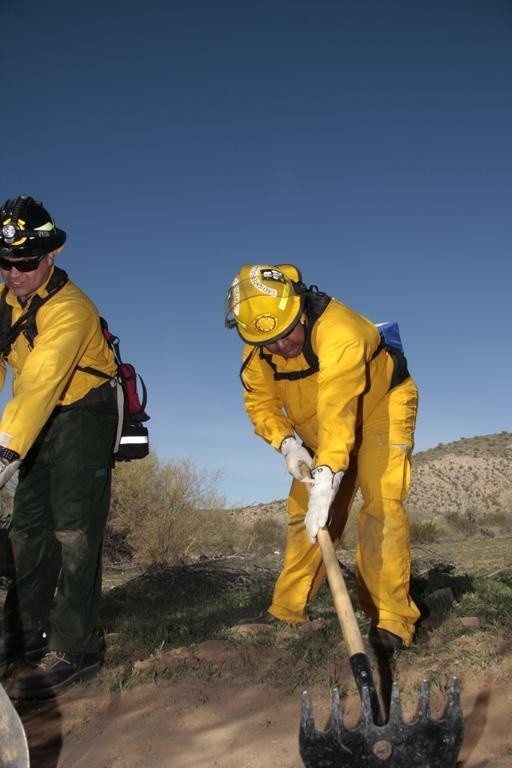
0 194 119 701
224 263 421 652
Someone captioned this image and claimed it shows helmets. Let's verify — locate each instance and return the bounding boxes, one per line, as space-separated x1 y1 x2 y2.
226 263 305 346
0 195 66 256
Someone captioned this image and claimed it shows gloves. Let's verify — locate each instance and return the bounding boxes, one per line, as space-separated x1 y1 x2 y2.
281 437 345 544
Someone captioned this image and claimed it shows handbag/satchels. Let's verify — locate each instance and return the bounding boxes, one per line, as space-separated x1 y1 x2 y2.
113 362 151 462
374 320 404 358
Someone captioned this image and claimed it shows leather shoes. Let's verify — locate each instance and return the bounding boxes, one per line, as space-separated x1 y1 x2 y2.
362 626 402 658
0 631 102 700
238 611 279 625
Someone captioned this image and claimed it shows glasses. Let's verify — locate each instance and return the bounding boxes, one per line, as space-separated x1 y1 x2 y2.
0 255 43 272
